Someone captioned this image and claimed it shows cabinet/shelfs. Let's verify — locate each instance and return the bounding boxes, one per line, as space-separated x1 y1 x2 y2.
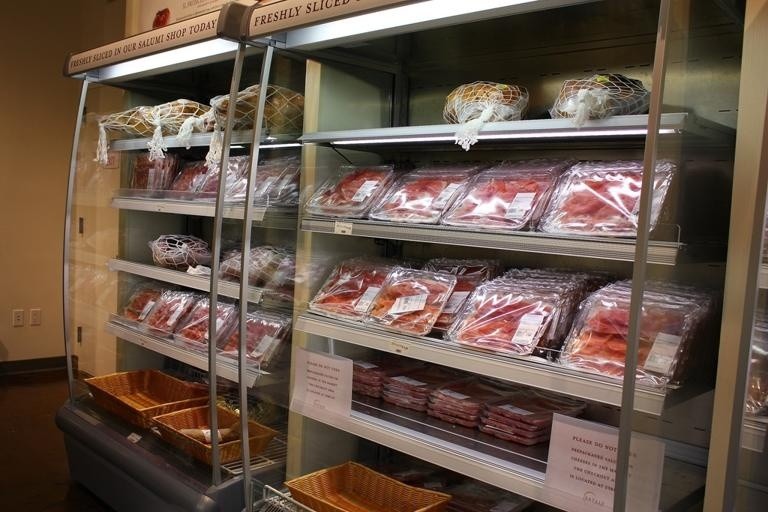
55 0 302 512
238 1 744 512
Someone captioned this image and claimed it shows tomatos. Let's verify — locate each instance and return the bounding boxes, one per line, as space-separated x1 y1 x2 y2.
152 8 170 27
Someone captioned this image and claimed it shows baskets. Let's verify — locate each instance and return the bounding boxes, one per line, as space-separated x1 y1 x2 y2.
151 402 280 466
82 363 212 431
283 458 453 512
